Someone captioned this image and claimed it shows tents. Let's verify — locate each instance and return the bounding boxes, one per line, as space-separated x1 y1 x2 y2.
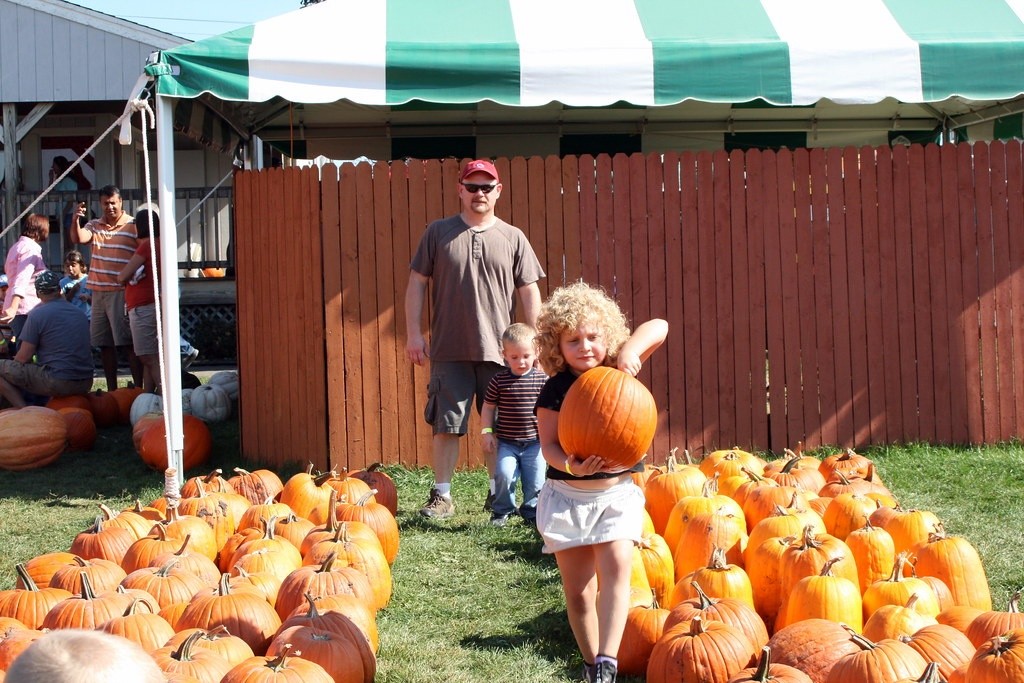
120 0 1024 470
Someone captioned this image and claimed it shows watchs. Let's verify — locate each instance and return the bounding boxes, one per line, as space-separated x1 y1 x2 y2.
481 427 493 435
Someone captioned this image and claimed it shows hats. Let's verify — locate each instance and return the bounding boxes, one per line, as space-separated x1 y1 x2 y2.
459 160 499 186
35 270 60 294
0 274 9 288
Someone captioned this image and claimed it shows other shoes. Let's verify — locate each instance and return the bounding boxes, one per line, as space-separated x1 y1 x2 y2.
182 347 199 369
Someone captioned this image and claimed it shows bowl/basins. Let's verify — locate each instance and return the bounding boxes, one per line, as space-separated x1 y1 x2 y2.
205 267 227 277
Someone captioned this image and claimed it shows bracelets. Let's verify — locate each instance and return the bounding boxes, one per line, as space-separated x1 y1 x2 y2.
566 456 585 478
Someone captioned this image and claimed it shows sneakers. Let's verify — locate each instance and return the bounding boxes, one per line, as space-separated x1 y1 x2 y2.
420 488 454 517
488 511 510 528
525 517 537 528
580 659 620 683
482 489 496 514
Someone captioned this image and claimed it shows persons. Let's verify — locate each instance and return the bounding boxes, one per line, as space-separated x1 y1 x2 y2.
3 628 166 683
47 153 97 259
74 184 199 393
533 282 668 683
404 161 546 518
481 323 547 526
0 215 92 408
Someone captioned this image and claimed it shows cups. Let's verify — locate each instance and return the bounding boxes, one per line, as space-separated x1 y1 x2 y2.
178 268 189 278
190 267 199 278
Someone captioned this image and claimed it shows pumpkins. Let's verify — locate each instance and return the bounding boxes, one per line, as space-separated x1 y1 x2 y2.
593 444 1024 683
0 370 238 473
558 366 657 474
0 460 398 683
202 268 224 277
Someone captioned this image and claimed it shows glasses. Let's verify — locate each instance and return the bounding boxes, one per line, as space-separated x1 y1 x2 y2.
459 182 497 193
